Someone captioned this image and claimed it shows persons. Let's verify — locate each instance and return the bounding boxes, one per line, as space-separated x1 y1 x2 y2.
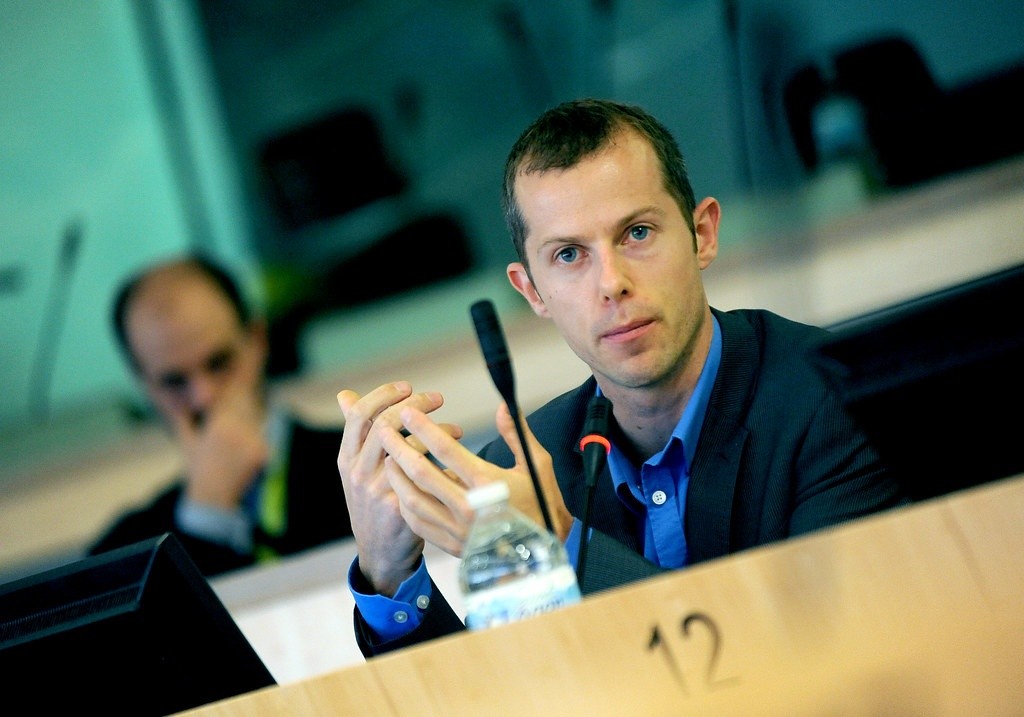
337 98 915 661
83 256 435 578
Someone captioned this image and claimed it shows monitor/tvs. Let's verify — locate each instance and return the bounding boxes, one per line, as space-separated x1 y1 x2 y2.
0 530 280 717
805 261 1023 504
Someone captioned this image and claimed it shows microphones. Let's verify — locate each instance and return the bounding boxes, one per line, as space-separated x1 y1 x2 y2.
25 226 82 427
470 298 555 535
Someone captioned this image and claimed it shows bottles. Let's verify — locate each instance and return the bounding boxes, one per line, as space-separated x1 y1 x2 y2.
461 482 582 632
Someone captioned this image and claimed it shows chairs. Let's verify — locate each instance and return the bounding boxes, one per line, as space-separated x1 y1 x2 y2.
781 35 968 190
256 103 421 272
